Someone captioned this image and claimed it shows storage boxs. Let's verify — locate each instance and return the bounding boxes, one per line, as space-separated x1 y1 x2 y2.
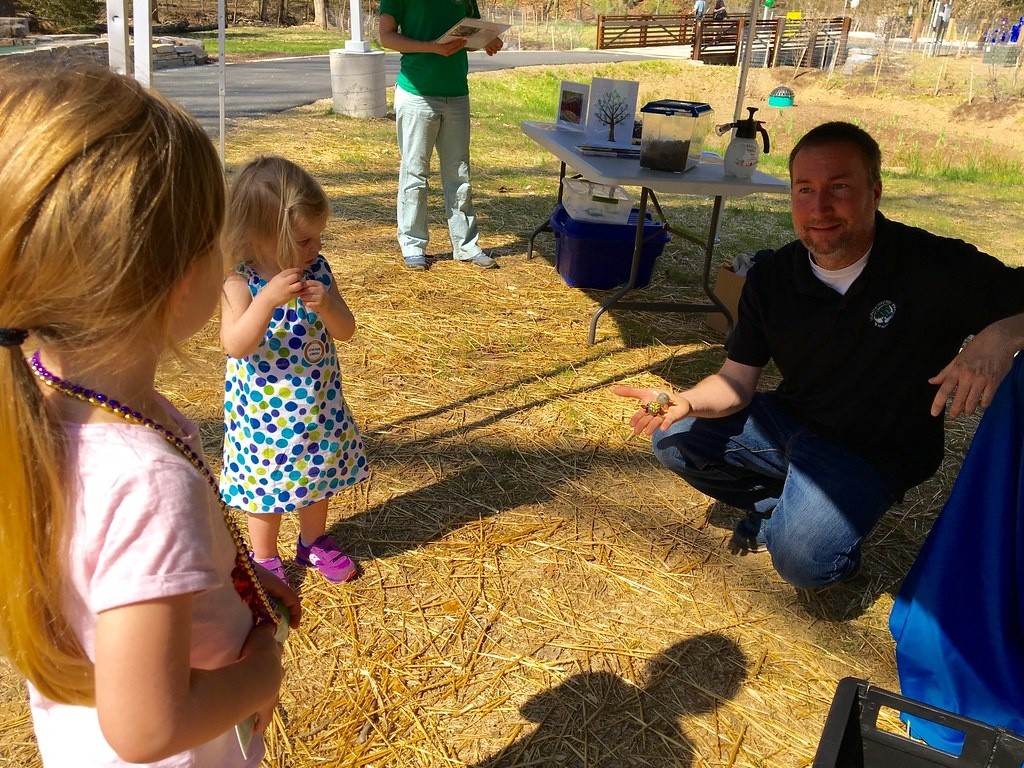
640 99 715 175
550 202 671 291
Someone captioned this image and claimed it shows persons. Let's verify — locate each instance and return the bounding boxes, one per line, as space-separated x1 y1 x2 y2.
608 122 1022 594
371 3 517 272
688 0 707 22
0 66 307 767
711 0 727 46
213 157 361 601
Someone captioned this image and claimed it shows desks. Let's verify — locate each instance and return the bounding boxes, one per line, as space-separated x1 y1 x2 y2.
519 121 791 346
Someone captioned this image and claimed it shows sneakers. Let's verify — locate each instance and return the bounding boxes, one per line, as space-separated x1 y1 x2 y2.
296 536 356 581
249 553 288 586
462 251 497 269
404 256 427 270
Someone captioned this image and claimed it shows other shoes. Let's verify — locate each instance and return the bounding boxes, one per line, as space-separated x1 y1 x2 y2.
733 517 771 553
841 547 862 583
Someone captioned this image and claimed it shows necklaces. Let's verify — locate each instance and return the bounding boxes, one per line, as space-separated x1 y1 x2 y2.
30 347 288 627
